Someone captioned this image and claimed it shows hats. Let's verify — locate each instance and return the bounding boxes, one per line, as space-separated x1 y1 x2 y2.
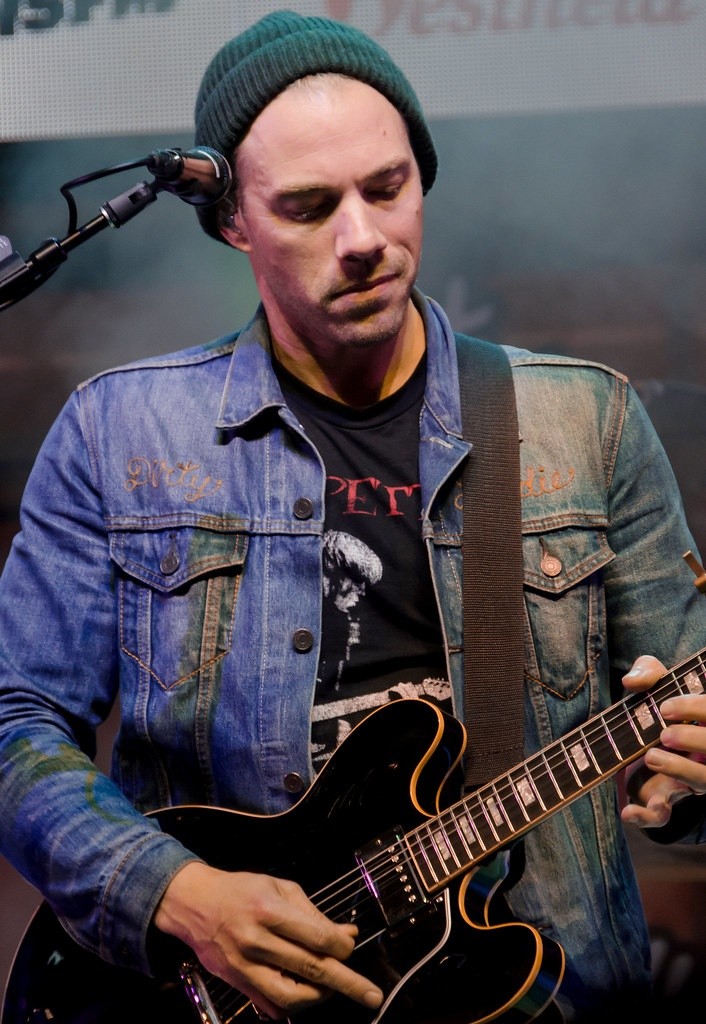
192 9 438 247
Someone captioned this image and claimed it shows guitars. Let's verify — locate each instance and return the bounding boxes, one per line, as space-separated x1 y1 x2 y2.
2 647 705 1024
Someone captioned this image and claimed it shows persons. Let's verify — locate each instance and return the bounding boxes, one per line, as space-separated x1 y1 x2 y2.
0 9 706 1024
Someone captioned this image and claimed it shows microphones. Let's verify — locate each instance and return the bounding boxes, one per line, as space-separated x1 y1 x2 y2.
148 145 233 207
